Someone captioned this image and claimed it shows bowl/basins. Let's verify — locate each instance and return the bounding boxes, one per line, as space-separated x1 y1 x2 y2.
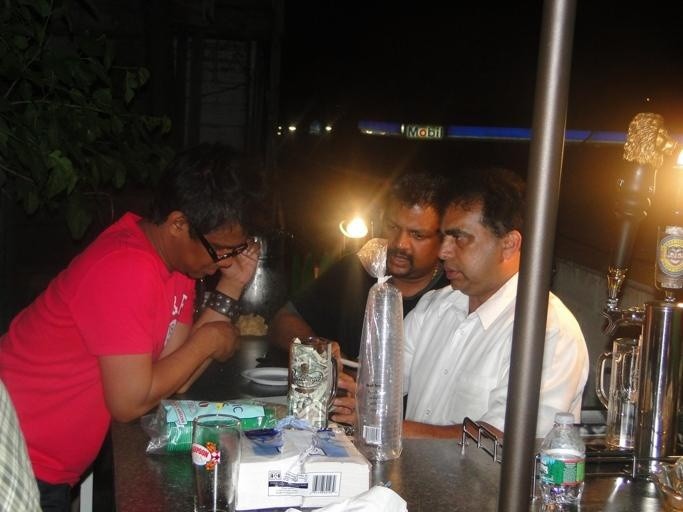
648 456 683 512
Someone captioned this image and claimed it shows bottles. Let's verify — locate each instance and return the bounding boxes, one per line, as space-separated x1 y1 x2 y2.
539 413 586 511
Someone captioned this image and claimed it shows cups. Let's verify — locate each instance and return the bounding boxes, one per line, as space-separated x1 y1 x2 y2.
192 414 241 512
595 337 641 448
161 408 278 453
361 284 403 463
287 336 338 431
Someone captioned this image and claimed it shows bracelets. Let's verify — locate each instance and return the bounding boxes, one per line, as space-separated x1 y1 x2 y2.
204 290 241 323
311 334 328 348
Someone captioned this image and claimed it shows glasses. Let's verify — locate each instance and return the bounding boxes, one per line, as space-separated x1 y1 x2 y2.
196 230 247 265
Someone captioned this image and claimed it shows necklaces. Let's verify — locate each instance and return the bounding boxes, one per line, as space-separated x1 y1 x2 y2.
382 266 439 285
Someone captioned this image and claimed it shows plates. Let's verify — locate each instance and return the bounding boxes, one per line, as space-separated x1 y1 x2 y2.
241 366 288 385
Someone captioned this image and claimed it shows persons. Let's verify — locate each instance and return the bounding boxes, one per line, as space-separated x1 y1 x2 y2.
263 170 455 431
328 167 594 433
0 163 260 512
